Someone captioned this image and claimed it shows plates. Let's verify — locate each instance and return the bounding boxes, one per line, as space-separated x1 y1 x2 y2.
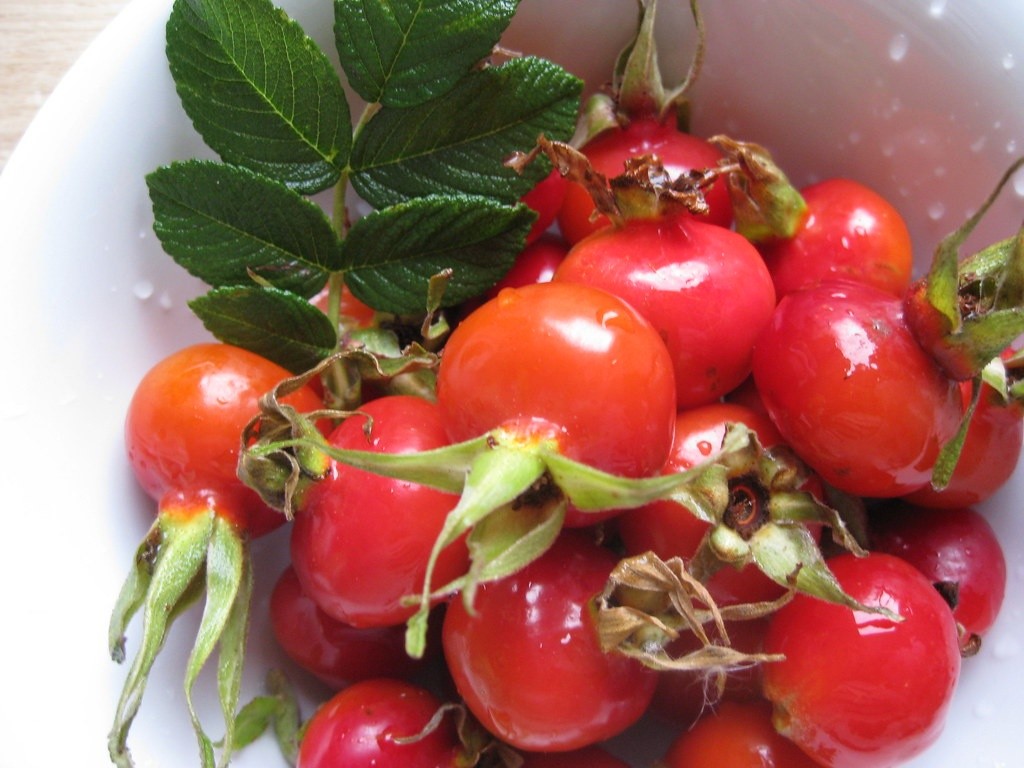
0 0 1024 768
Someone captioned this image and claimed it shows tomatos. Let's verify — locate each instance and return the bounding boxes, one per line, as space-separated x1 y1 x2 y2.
269 180 1024 768
